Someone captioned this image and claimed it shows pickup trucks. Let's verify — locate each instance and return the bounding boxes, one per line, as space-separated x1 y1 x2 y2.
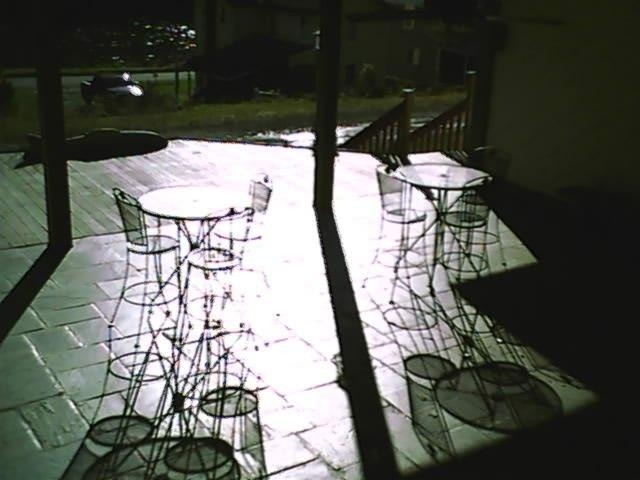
80 70 145 112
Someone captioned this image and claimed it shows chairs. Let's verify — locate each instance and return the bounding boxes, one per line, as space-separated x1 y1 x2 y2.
112 172 275 309
376 145 514 289
